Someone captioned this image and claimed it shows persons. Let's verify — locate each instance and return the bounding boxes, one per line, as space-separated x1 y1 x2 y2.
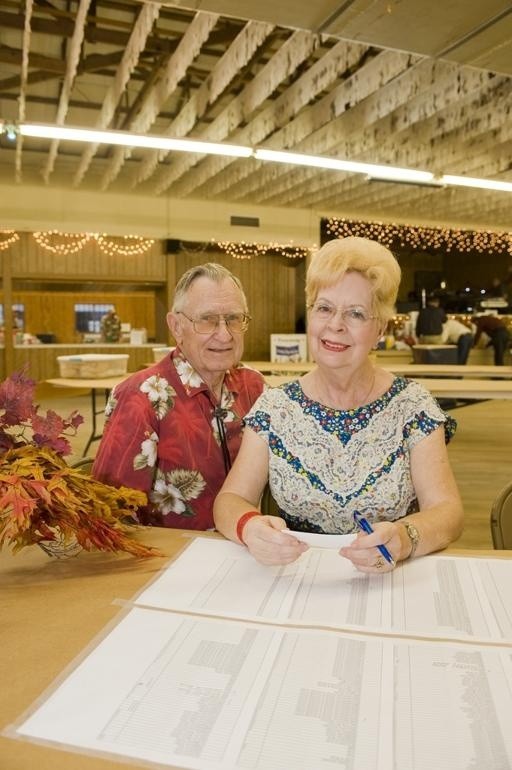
213 236 465 574
471 316 512 380
91 263 273 532
440 319 473 379
415 295 447 344
100 309 122 343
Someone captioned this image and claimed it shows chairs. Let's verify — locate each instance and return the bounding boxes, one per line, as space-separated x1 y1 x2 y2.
490 483 512 552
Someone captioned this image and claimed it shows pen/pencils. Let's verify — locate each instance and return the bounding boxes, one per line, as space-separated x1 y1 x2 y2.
353 510 396 567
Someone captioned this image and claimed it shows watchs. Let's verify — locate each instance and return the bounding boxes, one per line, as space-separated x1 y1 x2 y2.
396 520 420 561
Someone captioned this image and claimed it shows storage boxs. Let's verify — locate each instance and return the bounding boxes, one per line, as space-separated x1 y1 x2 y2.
152 347 176 363
57 353 129 379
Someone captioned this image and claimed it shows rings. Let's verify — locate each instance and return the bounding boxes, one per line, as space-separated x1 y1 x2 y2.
375 554 384 569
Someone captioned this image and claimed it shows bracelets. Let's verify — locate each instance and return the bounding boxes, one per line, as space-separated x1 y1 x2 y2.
237 511 263 547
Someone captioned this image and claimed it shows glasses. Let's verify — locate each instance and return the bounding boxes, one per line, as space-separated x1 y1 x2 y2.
307 302 381 329
175 311 252 334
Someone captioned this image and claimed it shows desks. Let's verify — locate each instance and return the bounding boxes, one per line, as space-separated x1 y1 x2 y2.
377 363 512 378
374 349 511 365
407 378 512 399
1 344 167 383
0 526 511 770
238 360 317 372
45 373 132 458
